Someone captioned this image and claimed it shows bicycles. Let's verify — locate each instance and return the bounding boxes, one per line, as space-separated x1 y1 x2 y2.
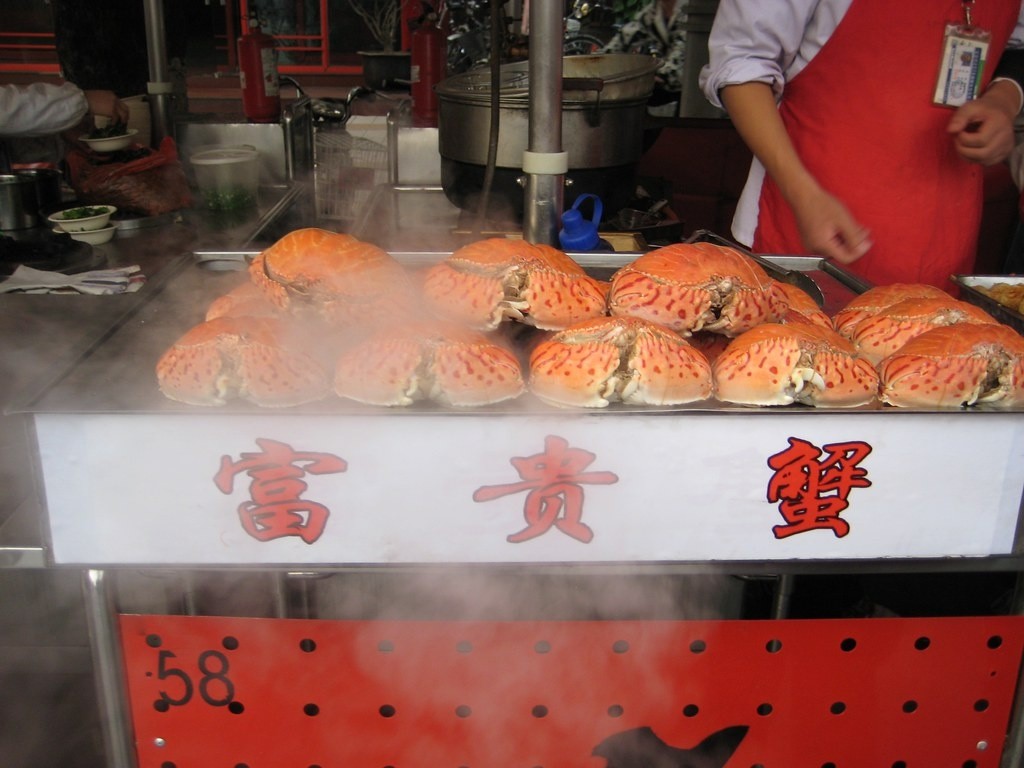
276 72 420 231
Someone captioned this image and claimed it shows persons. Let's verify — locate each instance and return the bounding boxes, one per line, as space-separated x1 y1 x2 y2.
709 0 1024 299
0 81 130 137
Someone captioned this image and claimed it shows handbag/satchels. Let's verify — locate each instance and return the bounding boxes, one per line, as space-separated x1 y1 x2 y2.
67 137 191 215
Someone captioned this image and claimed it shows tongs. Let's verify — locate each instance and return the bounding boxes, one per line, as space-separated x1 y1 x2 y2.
684 229 825 307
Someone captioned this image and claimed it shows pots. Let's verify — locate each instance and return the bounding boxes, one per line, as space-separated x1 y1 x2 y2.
439 77 649 168
437 53 666 102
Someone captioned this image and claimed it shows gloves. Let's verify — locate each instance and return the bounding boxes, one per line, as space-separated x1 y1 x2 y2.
0 264 146 295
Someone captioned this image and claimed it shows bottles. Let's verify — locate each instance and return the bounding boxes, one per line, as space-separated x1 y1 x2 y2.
559 193 614 254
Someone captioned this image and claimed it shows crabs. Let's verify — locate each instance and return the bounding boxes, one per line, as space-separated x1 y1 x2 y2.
153 226 1024 414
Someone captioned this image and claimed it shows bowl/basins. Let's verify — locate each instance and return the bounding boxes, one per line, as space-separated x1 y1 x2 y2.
53 225 118 244
79 128 138 152
48 205 117 231
189 148 260 211
